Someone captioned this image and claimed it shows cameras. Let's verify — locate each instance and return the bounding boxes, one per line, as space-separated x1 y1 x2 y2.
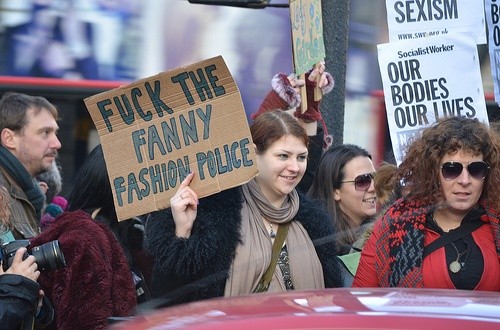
2 240 68 273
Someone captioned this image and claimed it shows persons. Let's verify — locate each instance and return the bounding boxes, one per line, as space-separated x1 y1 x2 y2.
29 145 149 330
0 92 62 240
251 62 334 196
308 144 378 288
0 186 53 330
352 119 500 292
149 109 343 306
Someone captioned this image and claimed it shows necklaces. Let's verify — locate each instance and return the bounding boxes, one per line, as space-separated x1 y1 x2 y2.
268 221 276 237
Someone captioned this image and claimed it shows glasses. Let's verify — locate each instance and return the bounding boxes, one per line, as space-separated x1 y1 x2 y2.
341 172 376 191
440 162 490 180
